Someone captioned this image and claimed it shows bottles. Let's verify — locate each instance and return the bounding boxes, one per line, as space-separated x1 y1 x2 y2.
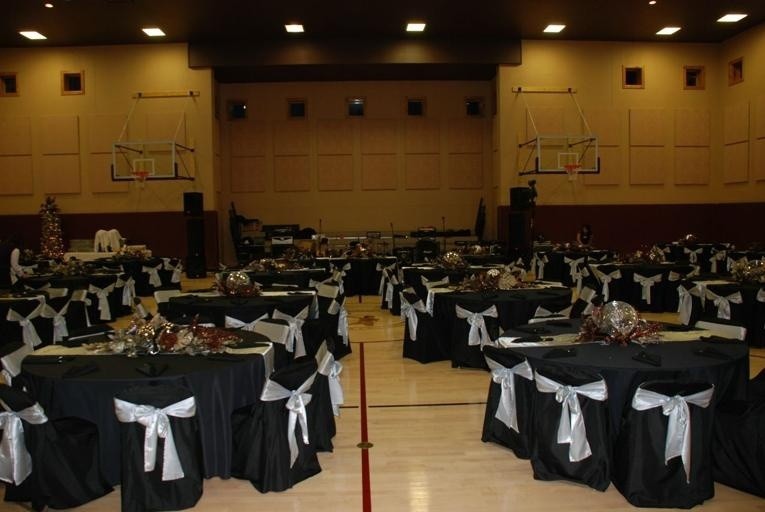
132 296 153 322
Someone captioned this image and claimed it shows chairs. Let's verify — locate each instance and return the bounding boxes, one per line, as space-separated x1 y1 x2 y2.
0 381 113 512
231 357 322 493
718 385 765 498
481 345 535 458
112 376 203 512
623 379 717 510
531 362 620 492
